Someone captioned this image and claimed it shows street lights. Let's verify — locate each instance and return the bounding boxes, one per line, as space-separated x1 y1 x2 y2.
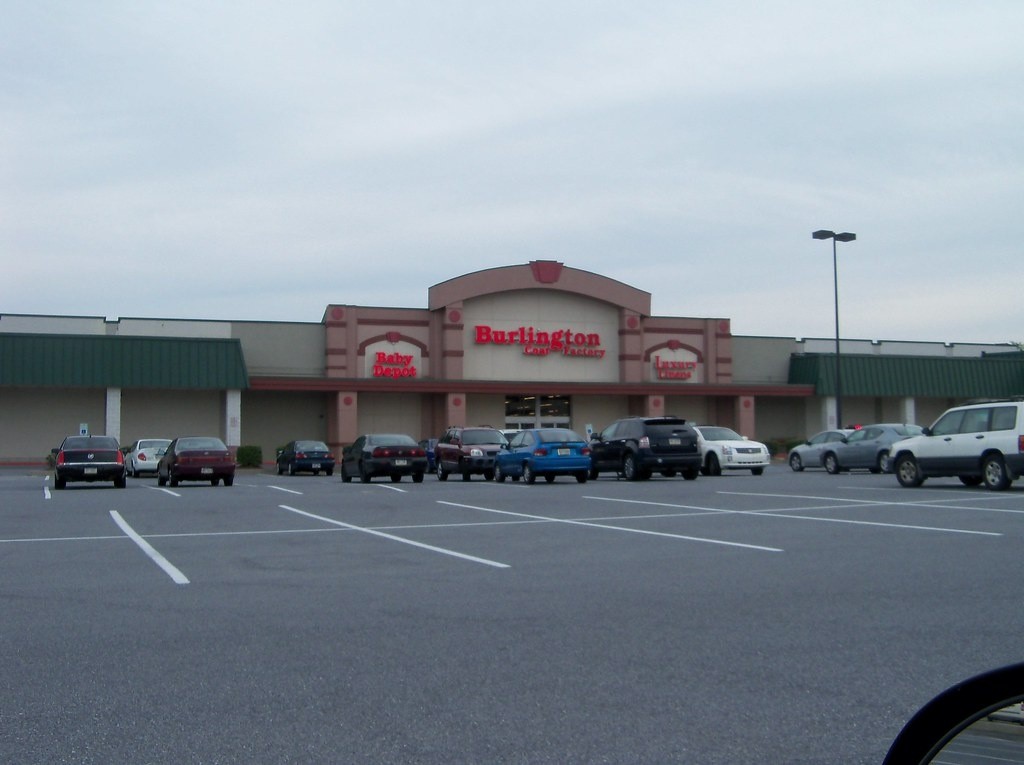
812 229 857 431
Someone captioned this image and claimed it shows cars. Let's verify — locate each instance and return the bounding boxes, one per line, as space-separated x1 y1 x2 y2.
51 435 120 488
986 702 1023 727
498 430 525 441
491 429 592 485
125 439 173 477
157 436 235 487
275 441 335 475
660 425 770 476
417 438 438 474
819 423 923 474
788 429 873 472
341 433 427 483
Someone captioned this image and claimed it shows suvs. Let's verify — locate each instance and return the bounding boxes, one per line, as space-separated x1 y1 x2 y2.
434 425 509 481
887 395 1024 491
586 416 703 481
